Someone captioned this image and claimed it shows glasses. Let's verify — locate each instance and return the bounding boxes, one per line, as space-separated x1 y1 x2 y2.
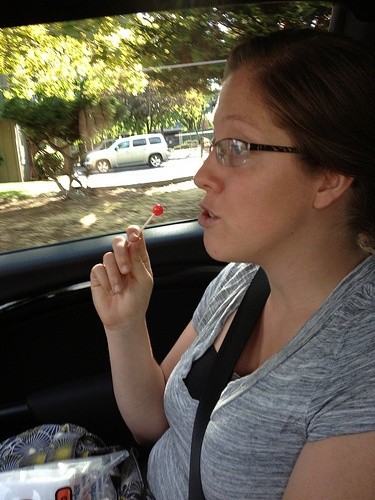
198 134 306 167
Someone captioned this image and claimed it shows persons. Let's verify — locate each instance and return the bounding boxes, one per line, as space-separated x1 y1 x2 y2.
0 25 375 499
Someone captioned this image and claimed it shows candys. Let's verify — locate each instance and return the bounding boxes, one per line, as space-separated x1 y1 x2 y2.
153 204 164 217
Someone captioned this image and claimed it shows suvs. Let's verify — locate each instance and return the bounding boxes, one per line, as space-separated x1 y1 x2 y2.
83 134 170 175
